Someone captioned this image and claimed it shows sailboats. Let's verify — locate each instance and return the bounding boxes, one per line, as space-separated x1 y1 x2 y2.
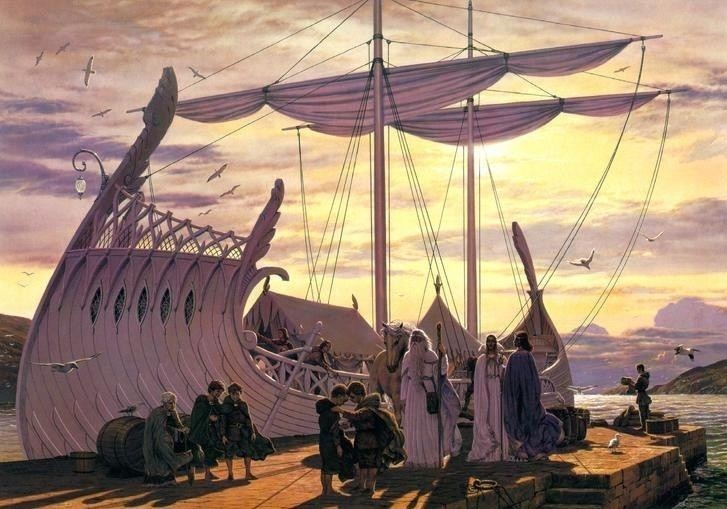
13 0 688 463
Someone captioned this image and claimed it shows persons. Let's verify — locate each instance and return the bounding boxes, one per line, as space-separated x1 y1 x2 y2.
315 382 394 497
401 327 546 469
629 362 652 430
142 380 258 484
262 327 339 395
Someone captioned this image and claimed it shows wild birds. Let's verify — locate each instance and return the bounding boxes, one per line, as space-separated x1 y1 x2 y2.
613 65 631 74
31 39 96 87
567 247 596 272
28 351 105 374
639 230 664 242
90 107 112 119
199 163 243 218
566 384 598 394
118 400 147 417
16 270 36 286
188 67 207 82
673 340 700 362
608 433 623 454
